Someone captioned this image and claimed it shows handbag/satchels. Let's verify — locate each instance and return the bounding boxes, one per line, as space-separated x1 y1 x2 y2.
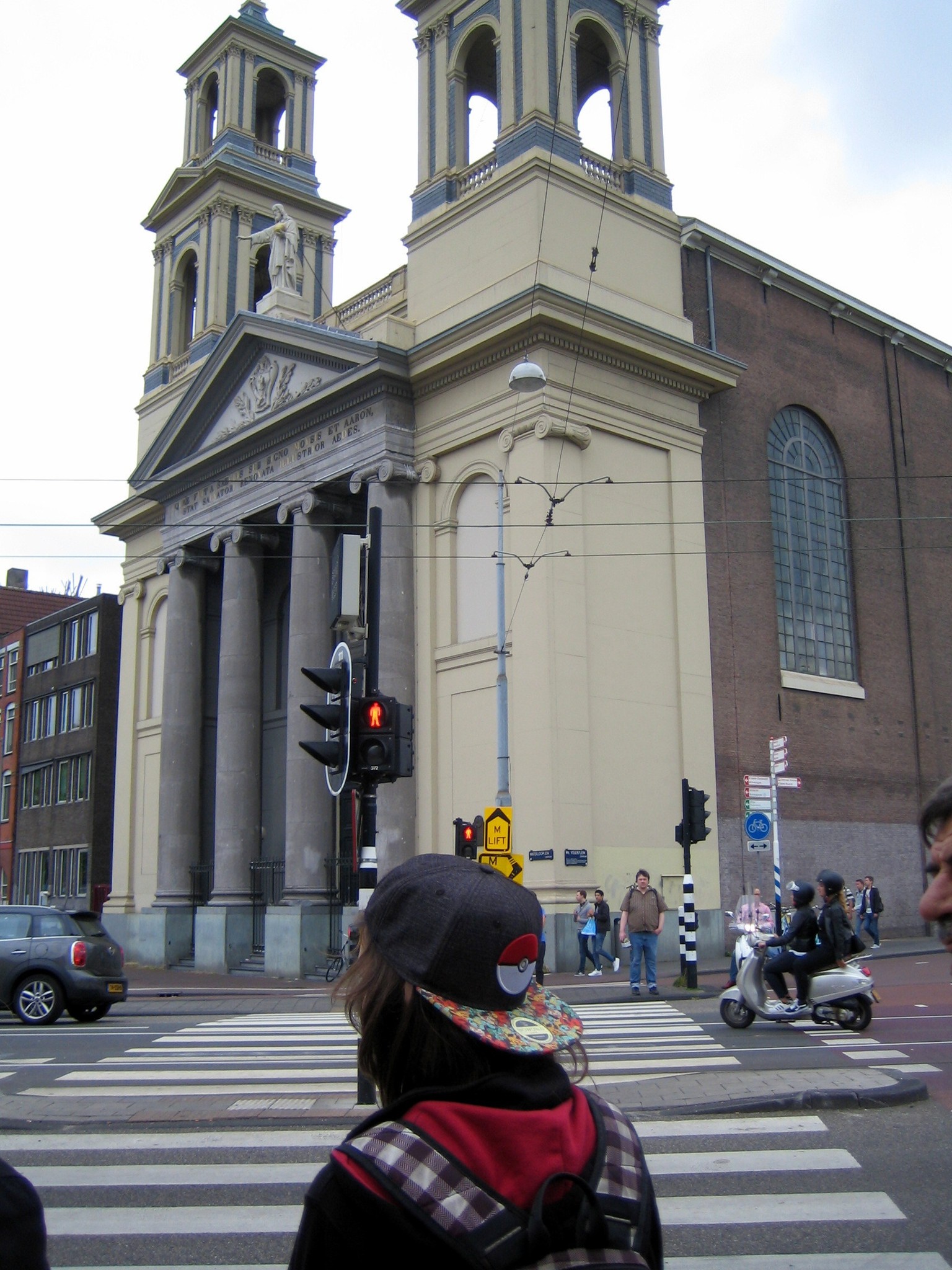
581 916 596 935
833 934 866 957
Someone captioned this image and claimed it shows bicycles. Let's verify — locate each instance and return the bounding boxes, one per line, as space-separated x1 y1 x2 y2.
325 929 357 983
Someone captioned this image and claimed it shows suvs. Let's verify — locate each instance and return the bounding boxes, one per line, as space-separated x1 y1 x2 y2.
0 902 130 1026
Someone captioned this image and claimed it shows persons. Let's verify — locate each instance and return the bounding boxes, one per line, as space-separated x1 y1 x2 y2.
758 880 819 1013
843 876 882 949
573 890 603 977
784 870 855 1016
0 1158 52 1270
235 204 299 292
620 869 669 996
535 909 547 986
916 777 952 951
587 890 620 976
721 888 782 989
288 853 666 1270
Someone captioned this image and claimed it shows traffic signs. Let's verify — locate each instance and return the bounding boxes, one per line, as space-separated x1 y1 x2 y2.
773 748 788 762
743 774 771 788
773 736 787 750
774 760 788 775
744 798 772 811
776 776 802 789
743 786 771 799
744 810 772 823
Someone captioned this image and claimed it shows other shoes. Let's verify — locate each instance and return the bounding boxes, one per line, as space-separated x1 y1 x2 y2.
588 969 602 977
594 961 603 970
870 943 881 949
612 958 620 972
632 987 641 996
721 980 736 989
649 986 659 995
574 971 585 977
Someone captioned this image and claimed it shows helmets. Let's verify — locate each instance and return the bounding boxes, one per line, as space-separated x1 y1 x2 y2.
816 869 844 896
792 880 815 903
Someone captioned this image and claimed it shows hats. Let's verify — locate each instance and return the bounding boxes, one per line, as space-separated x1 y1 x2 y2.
365 853 583 1055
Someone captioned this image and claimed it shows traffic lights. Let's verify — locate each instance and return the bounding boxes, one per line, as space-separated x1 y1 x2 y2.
354 696 398 781
459 822 477 860
688 789 712 845
297 640 353 797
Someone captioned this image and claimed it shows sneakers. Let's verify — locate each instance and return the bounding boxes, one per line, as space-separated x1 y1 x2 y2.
775 998 795 1005
768 1003 792 1013
783 998 809 1015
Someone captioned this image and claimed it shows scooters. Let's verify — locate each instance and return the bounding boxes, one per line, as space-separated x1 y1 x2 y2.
724 894 777 973
718 927 881 1031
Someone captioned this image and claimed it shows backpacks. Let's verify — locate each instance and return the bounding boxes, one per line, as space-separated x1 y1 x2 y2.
873 888 884 912
845 888 855 908
336 1085 654 1270
